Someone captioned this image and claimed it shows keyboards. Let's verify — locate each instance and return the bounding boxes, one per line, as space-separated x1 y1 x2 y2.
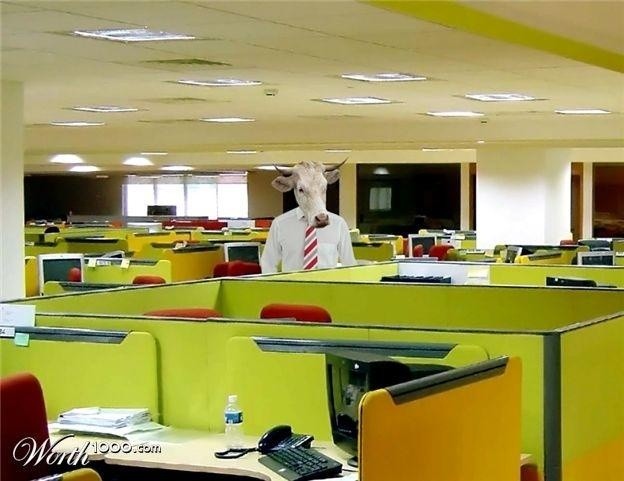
258 446 342 481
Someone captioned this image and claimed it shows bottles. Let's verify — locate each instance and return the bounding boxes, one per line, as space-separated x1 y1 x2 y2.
225 395 243 449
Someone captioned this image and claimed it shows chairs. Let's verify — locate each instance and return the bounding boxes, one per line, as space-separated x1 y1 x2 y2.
0 372 55 481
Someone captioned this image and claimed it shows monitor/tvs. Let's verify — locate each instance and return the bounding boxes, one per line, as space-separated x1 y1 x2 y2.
325 347 455 468
577 251 616 266
546 277 597 287
408 234 437 258
38 252 85 297
224 242 261 265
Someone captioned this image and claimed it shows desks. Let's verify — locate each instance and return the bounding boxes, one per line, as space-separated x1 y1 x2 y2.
44 416 359 480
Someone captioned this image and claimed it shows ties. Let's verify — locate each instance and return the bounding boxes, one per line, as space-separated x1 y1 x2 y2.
304 222 317 272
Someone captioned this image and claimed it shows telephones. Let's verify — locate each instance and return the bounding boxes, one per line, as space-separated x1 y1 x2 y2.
258 425 315 453
